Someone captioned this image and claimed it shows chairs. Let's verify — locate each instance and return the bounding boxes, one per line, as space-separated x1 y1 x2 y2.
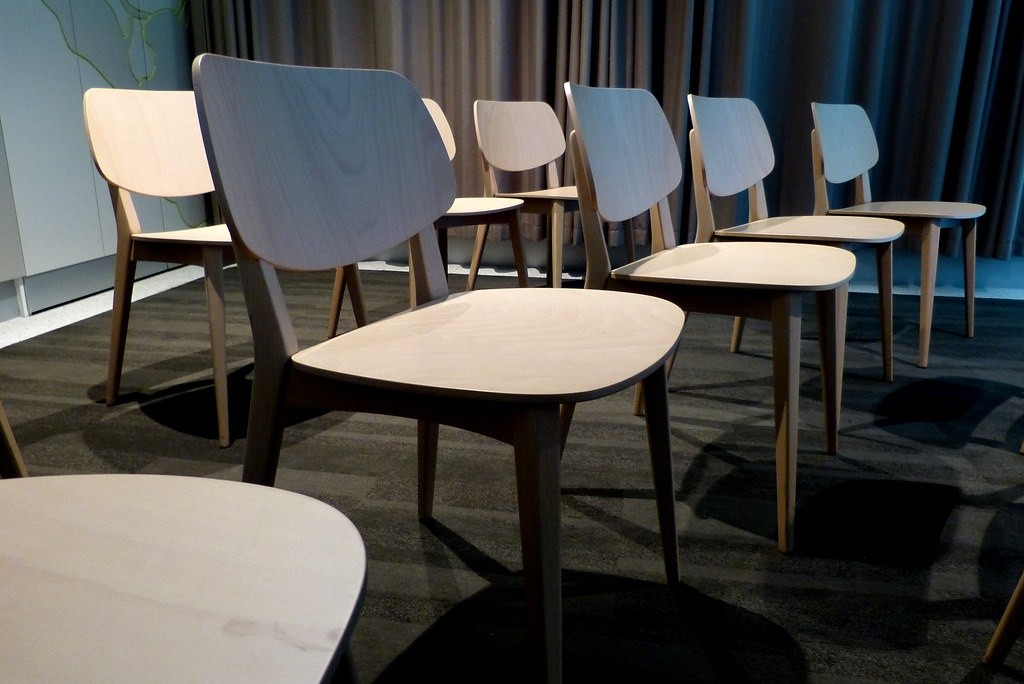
84 86 367 449
192 52 686 684
326 98 527 340
664 93 907 458
809 102 986 368
561 83 856 550
465 100 589 293
0 473 365 684
